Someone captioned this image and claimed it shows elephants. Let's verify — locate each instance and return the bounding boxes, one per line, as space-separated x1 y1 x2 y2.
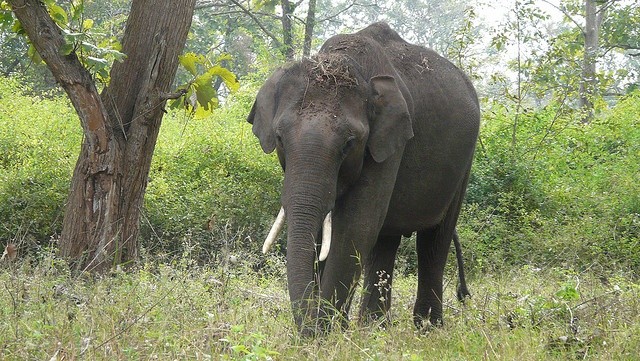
246 20 481 346
